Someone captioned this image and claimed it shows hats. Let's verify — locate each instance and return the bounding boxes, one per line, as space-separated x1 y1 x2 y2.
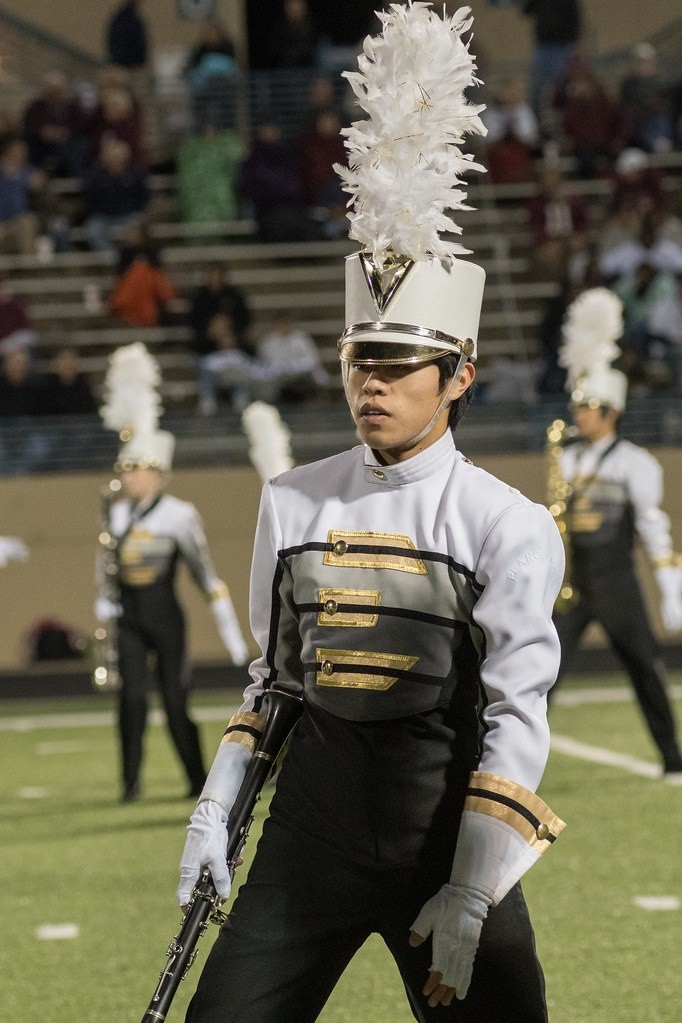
96 341 177 475
556 286 628 413
332 0 487 365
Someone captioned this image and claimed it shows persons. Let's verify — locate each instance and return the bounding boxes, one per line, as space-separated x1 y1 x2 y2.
545 371 682 781
96 432 248 801
0 0 681 462
175 246 568 1023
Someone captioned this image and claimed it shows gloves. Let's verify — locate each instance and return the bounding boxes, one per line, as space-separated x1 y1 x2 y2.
207 582 250 665
654 565 682 629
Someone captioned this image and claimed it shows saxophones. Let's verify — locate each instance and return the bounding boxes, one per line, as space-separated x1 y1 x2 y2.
91 480 124 698
541 419 583 620
